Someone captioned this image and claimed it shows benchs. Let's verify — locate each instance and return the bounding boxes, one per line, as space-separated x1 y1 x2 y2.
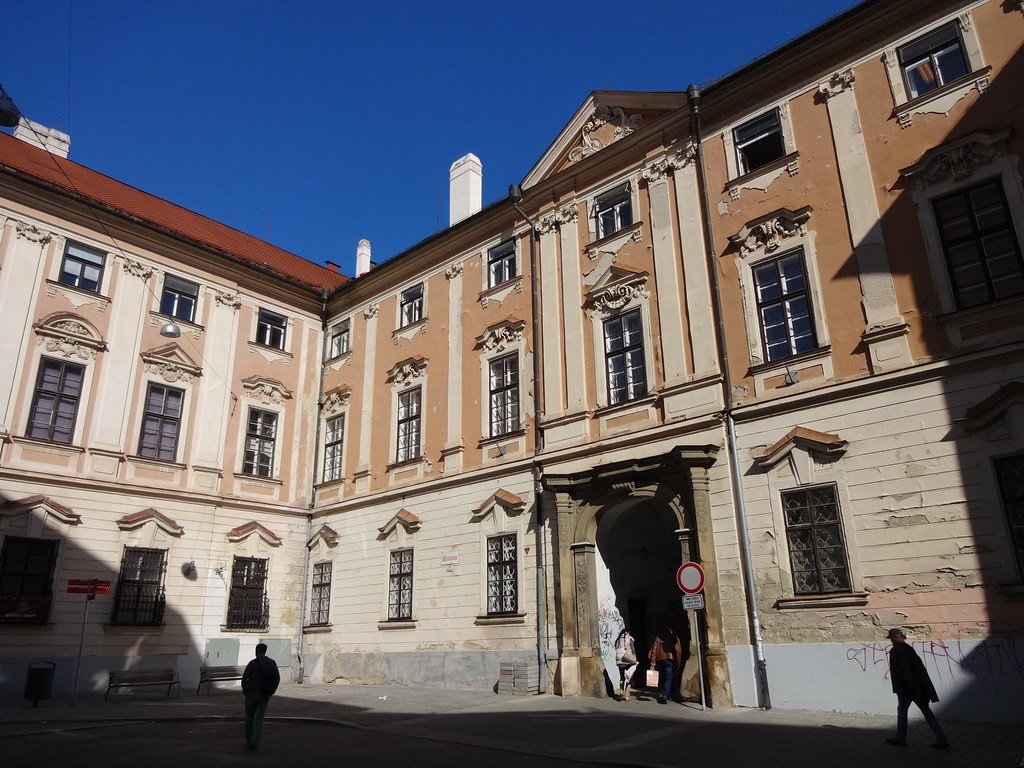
105 668 181 703
197 665 248 697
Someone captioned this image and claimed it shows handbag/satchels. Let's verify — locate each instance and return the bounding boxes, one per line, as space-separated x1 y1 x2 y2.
646 666 659 687
622 652 638 664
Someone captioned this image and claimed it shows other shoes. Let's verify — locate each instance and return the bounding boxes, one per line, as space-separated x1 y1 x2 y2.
658 695 667 704
931 741 951 749
245 742 258 751
619 691 633 702
885 737 906 745
669 694 676 700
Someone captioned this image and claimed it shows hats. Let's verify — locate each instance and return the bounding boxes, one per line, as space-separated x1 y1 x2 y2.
886 629 902 639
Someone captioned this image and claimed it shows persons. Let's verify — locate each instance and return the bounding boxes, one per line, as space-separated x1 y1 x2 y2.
885 629 952 750
242 643 280 751
614 628 636 702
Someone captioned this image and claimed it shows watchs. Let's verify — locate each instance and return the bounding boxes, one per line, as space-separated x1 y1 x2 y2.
647 623 682 704
